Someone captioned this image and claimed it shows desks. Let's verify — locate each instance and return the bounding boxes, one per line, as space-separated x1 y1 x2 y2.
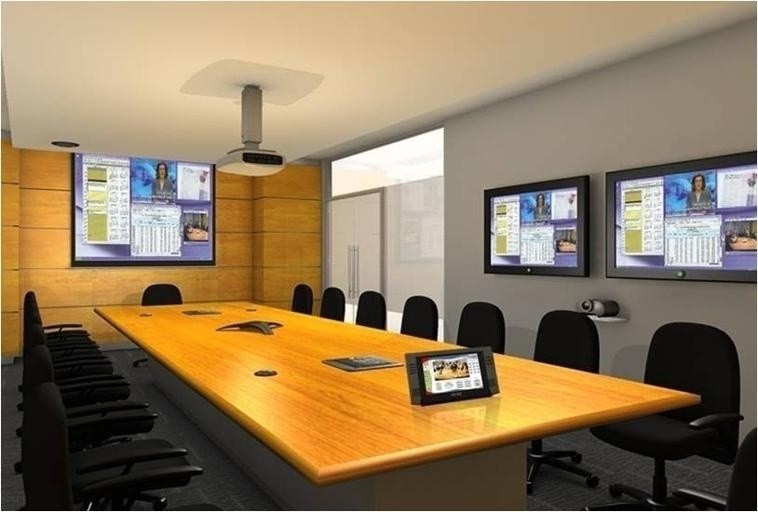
90 302 702 511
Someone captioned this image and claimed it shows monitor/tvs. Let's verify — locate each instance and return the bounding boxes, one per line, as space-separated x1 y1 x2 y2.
481 175 591 277
605 150 756 284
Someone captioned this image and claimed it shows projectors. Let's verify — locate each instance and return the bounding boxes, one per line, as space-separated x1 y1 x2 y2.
215 151 288 179
580 298 619 318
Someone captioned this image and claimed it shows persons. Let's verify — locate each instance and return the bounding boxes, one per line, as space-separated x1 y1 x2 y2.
533 193 548 218
687 175 711 208
152 164 174 195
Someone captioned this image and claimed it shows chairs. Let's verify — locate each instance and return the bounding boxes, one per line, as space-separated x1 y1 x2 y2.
319 287 346 323
26 384 191 505
455 300 507 357
586 320 745 512
133 284 184 369
526 310 599 495
355 290 387 331
19 290 157 462
13 456 202 511
291 284 314 315
400 295 440 342
